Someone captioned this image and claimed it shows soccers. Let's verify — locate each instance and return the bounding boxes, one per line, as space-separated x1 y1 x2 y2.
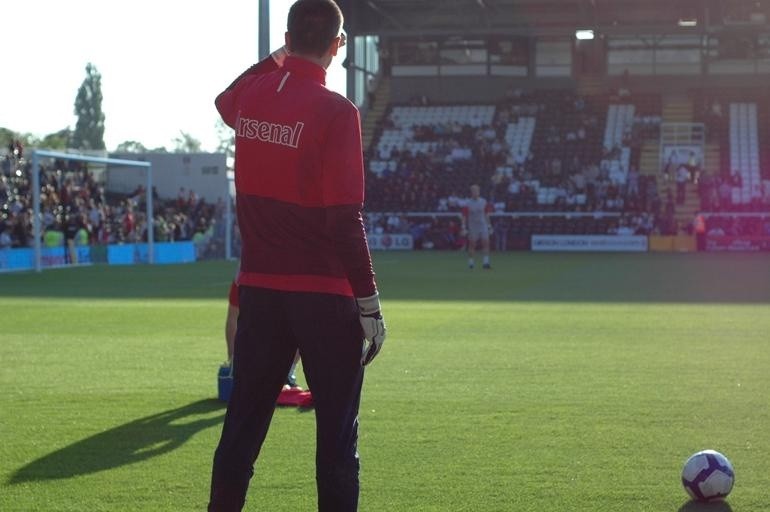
681 450 737 506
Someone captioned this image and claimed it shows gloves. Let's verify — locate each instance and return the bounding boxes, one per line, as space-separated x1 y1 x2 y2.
357 301 385 364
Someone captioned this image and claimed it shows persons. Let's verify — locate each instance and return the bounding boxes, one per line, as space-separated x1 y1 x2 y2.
360 77 769 256
224 272 313 409
0 136 242 266
209 1 387 511
458 181 495 273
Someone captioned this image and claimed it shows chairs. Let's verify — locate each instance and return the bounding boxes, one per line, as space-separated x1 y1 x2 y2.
357 82 769 251
